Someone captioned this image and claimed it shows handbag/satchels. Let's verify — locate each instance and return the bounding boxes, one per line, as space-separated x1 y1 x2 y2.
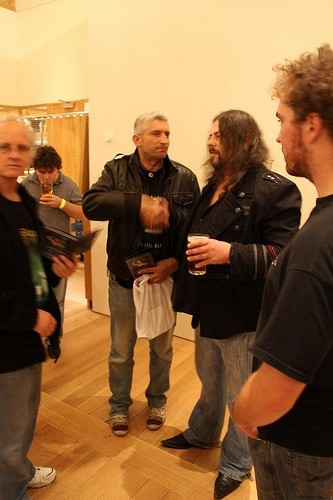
133 272 178 341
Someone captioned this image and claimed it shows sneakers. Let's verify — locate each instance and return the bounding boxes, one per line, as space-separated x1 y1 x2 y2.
28 466 56 488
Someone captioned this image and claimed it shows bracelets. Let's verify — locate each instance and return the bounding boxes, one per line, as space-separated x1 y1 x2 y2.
59 199 65 209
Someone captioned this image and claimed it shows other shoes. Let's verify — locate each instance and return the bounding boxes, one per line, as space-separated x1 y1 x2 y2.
146 403 167 431
108 414 129 436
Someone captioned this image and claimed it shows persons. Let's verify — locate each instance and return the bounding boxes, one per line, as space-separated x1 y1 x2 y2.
0 120 56 500
19 144 83 359
233 43 333 500
161 110 302 500
81 112 200 437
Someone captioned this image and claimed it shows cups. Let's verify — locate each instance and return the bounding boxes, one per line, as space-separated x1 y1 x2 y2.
41 182 53 197
187 232 209 275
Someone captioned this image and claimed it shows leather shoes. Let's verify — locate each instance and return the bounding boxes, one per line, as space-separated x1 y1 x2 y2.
161 432 197 449
214 472 242 500
